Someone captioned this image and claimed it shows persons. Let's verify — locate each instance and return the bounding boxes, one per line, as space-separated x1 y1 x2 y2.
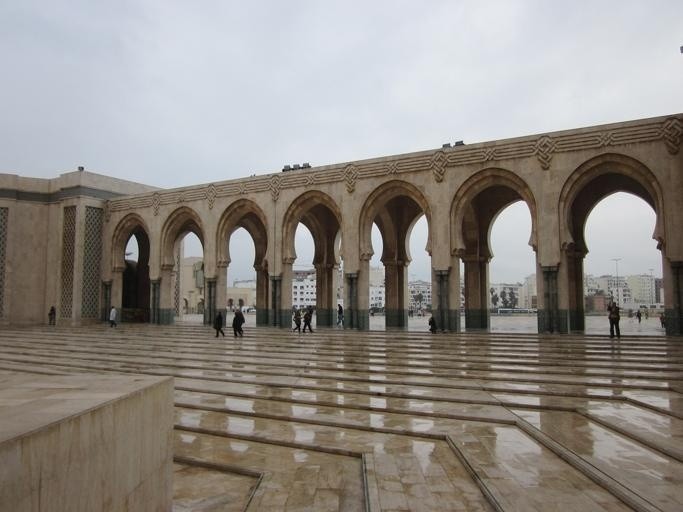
235 308 245 334
51 306 55 325
231 312 243 338
606 300 621 339
109 306 117 328
660 313 665 328
291 305 313 335
212 310 226 338
429 317 436 334
47 310 52 325
337 304 344 325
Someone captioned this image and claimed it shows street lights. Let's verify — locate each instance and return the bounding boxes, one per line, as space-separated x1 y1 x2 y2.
647 267 655 303
611 258 623 310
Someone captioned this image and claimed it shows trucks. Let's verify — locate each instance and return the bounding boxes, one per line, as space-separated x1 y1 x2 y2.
241 305 256 313
